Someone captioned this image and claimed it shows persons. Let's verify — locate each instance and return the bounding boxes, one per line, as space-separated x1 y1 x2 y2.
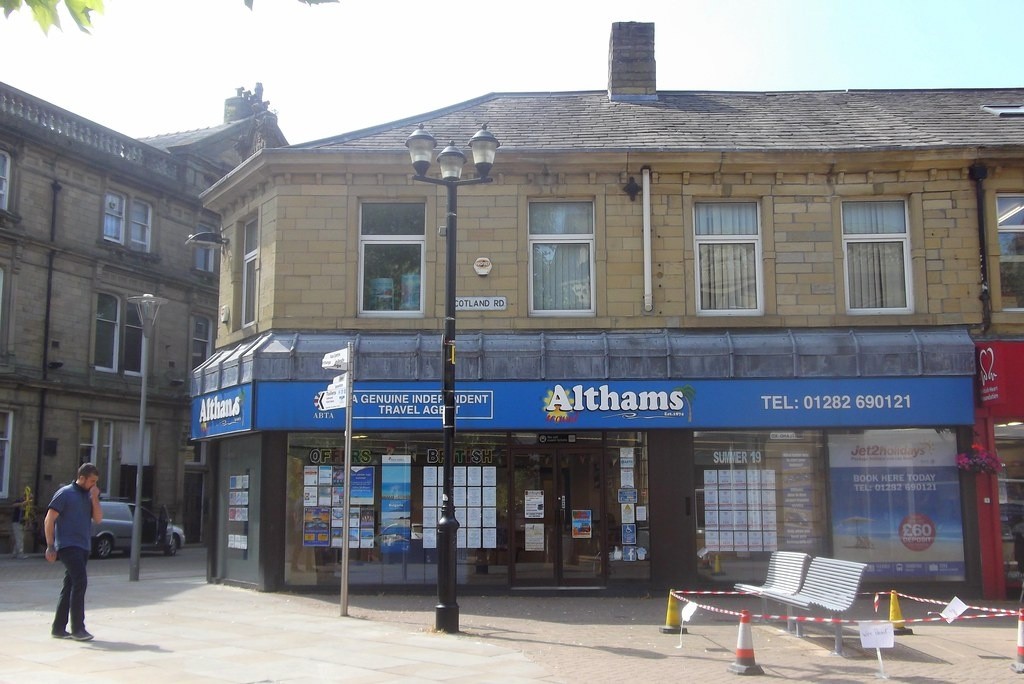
1012 516 1024 574
44 463 102 641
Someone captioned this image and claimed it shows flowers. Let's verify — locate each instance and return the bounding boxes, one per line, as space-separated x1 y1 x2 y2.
955 443 1010 475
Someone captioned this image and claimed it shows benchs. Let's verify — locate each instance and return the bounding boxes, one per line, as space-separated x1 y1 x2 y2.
734 551 869 656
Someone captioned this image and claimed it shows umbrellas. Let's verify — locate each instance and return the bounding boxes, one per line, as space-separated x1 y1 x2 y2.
842 517 876 545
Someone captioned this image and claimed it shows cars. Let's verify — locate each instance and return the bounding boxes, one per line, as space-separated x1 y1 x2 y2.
357 518 438 565
589 525 650 581
89 493 185 560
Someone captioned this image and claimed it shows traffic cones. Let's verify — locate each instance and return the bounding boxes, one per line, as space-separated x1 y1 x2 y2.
889 589 914 635
725 609 765 676
1009 607 1024 674
658 588 689 635
697 553 712 571
710 554 727 576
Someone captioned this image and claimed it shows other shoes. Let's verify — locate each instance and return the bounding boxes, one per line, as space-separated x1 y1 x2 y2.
52 631 73 639
71 630 94 641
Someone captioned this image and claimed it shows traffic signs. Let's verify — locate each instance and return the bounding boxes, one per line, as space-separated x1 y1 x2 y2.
313 388 347 411
327 372 348 390
321 347 348 371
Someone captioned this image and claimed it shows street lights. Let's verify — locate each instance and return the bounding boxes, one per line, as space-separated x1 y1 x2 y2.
123 293 171 582
403 122 501 634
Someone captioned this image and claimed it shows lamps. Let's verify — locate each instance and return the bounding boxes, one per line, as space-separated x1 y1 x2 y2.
185 232 230 250
